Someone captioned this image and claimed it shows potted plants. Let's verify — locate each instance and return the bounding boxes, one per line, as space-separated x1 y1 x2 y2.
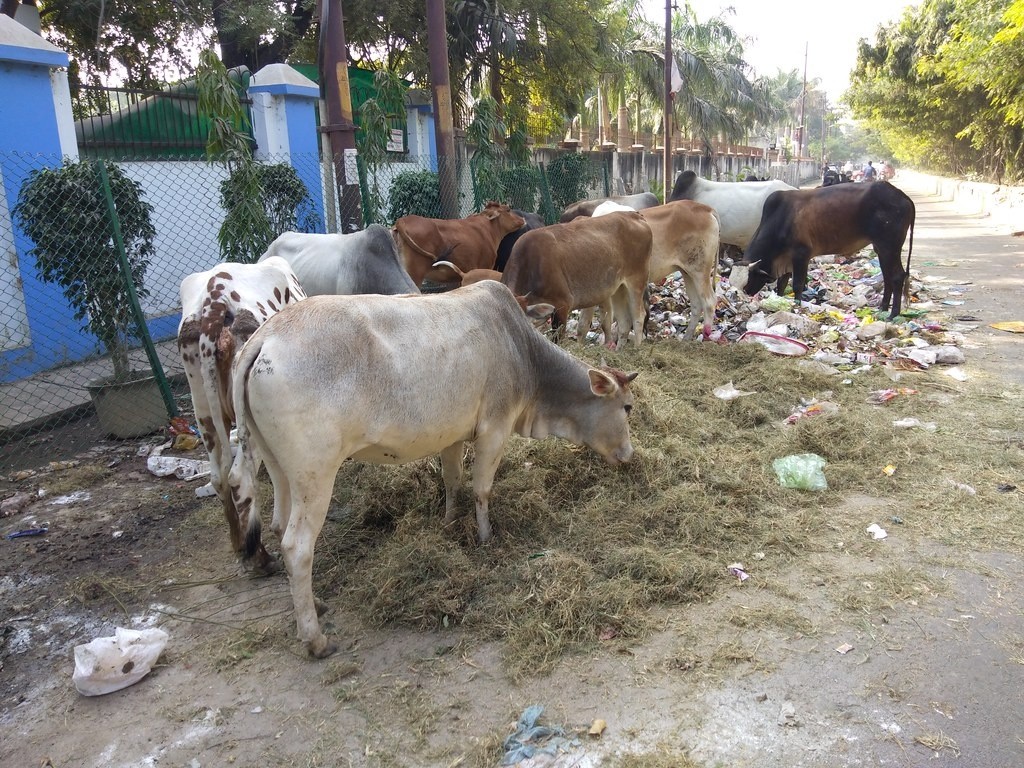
11 158 171 440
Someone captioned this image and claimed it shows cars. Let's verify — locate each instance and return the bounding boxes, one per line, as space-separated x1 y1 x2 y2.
851 164 895 181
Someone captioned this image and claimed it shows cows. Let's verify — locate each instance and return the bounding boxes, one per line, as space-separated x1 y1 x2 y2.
177 169 916 660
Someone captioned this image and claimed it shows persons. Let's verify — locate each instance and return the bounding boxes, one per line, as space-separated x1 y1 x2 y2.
823 159 894 181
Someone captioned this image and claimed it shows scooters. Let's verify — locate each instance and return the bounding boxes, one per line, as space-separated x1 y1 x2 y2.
878 170 888 183
862 176 876 183
